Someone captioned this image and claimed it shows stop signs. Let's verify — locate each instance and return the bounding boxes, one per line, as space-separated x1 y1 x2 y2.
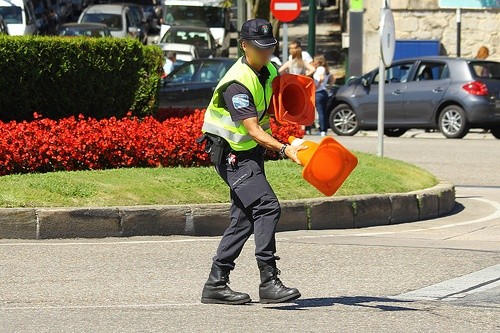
270 0 301 22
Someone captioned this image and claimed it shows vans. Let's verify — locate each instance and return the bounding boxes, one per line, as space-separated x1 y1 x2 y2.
325 56 500 139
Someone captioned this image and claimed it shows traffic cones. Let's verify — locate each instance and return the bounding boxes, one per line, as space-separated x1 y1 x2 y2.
287 135 358 196
268 74 316 126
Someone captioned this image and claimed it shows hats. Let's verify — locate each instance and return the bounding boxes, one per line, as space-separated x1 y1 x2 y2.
241 18 278 49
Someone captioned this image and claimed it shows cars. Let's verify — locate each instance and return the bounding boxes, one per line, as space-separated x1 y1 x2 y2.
155 57 281 119
0 0 231 71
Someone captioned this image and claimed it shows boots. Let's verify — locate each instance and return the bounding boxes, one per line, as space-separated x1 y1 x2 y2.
201 265 252 304
257 258 301 304
